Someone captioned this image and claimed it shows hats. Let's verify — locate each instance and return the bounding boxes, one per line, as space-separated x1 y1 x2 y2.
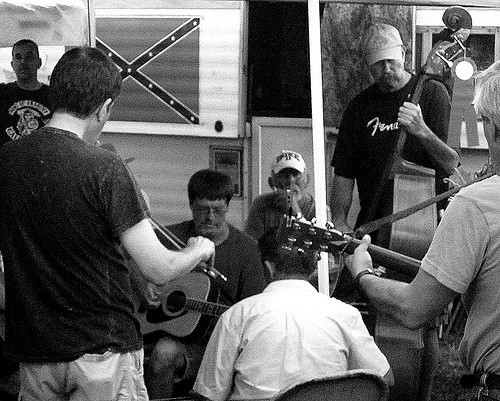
361 24 403 69
272 150 306 175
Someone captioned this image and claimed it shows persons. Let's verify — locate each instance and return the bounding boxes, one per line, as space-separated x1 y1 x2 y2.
193 224 396 401
345 59 500 401
124 168 268 401
0 38 216 401
244 150 331 279
331 23 460 401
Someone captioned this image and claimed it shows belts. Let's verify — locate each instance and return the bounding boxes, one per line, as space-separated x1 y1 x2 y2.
460 372 500 388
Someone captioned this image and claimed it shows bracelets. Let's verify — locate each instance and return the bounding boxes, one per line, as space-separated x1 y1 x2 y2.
355 268 377 285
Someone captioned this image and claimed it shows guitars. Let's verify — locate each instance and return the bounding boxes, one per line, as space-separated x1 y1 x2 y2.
130 269 234 338
328 5 473 401
276 213 471 317
